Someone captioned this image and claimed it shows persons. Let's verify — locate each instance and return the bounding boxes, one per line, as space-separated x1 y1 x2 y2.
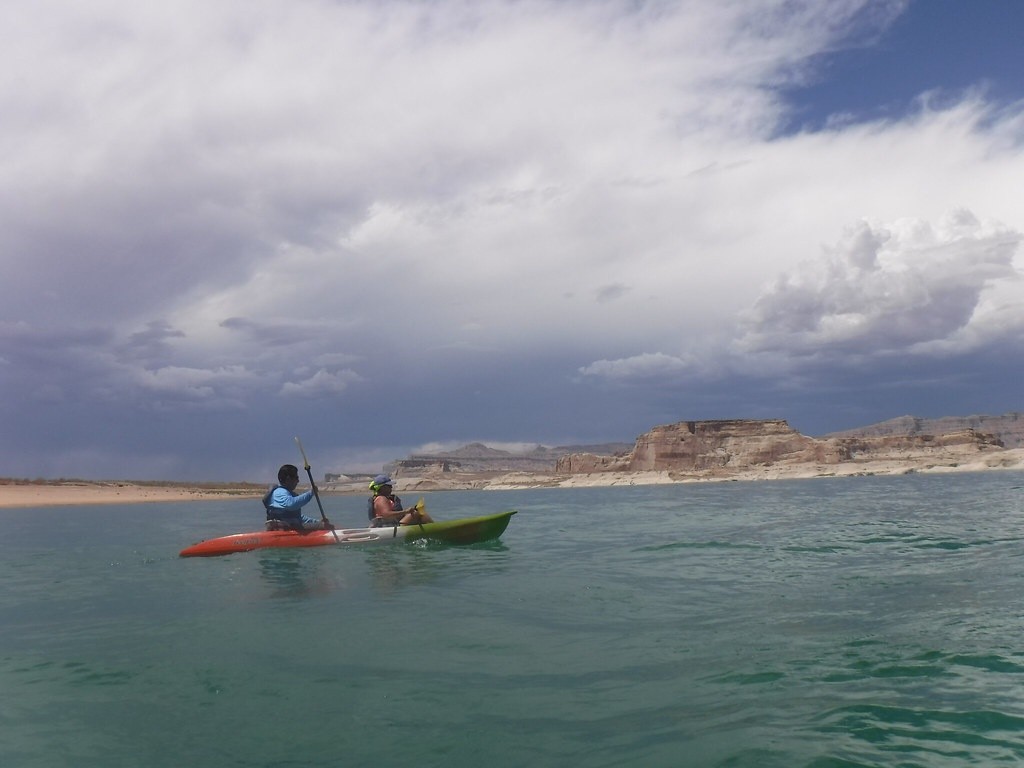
261 465 333 530
367 474 434 527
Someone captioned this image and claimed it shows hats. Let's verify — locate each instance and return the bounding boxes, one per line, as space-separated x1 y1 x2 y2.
373 475 397 485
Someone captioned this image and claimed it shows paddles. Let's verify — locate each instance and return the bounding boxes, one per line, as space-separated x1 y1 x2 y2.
412 497 426 517
293 435 333 530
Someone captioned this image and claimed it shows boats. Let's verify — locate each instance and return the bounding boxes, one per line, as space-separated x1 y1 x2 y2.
179 511 519 557
